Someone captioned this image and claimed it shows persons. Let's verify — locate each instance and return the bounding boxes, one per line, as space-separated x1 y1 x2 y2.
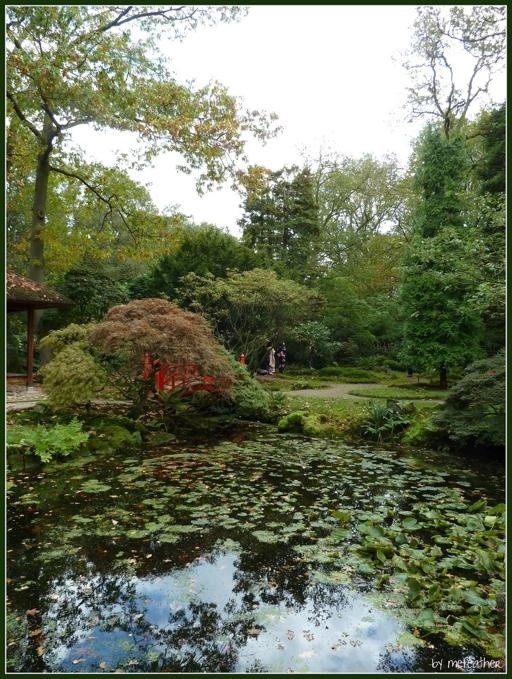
266 341 276 375
277 341 288 372
